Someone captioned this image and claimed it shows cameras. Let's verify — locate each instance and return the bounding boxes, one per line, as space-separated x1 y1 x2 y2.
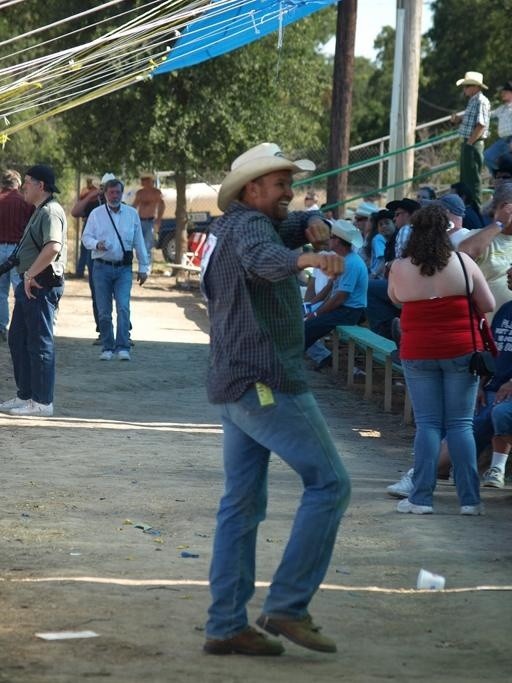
0 255 20 275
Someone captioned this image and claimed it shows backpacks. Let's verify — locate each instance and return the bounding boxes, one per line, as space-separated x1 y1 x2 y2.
469 348 499 377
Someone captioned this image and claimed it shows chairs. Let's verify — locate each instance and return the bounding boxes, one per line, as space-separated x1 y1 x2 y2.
167 233 207 293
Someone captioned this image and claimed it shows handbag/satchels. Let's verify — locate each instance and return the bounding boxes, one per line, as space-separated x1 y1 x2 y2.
44 260 65 288
123 248 135 264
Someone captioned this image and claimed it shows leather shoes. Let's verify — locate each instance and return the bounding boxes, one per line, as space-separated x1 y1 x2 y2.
203 622 287 658
255 606 339 654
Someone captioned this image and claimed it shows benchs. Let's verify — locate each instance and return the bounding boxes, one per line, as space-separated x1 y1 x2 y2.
481 187 494 205
331 324 413 426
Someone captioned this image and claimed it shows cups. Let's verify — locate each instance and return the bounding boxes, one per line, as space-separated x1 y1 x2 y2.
414 568 445 591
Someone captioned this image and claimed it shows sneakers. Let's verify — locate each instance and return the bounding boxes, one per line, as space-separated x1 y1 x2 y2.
385 466 416 498
8 398 56 417
395 496 434 515
69 273 85 278
458 500 489 516
93 334 104 345
482 465 506 488
128 338 135 346
0 395 33 414
0 329 9 344
99 349 113 360
117 350 131 360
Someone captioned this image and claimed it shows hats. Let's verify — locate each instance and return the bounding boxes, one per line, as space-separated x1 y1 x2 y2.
384 195 422 215
454 68 491 91
139 171 156 181
26 163 64 194
502 81 512 92
375 209 393 226
497 150 512 173
437 191 468 217
344 200 381 217
91 171 117 192
214 140 319 212
331 218 365 248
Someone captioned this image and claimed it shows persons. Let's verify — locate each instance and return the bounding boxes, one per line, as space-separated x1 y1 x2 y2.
131 174 165 265
72 173 134 347
200 141 355 658
1 166 66 420
450 71 511 215
303 185 511 518
1 168 36 335
82 179 149 361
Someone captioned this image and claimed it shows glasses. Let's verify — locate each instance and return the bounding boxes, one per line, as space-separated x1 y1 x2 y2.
394 210 408 216
495 175 512 180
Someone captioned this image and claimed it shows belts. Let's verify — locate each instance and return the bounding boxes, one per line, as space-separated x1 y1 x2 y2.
0 241 19 245
140 216 155 220
93 256 126 267
